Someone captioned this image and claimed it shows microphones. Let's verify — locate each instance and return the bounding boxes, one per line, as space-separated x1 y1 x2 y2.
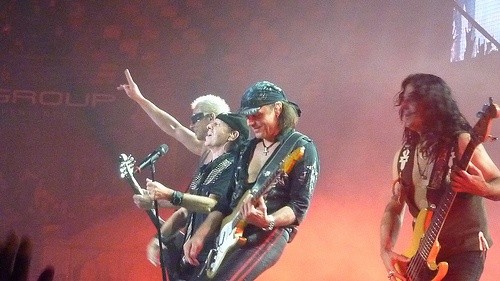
136 144 169 173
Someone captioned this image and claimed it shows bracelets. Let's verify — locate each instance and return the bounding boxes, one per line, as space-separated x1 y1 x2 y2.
170 190 183 206
155 232 167 243
262 215 274 231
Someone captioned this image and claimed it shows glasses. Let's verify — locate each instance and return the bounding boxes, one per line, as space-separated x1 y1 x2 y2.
191 112 212 124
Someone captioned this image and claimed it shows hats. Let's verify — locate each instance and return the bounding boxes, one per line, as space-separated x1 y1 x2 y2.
215 112 250 140
237 81 288 117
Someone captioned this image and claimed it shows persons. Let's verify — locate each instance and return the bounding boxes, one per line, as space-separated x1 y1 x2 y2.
381 73 500 281
147 113 250 281
116 69 231 209
183 81 320 281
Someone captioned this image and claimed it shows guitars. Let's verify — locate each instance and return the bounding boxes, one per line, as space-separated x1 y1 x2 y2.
203 145 306 281
392 96 500 281
119 152 187 281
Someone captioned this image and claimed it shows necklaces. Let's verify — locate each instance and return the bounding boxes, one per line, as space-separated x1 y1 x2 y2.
262 140 276 156
416 142 431 180
